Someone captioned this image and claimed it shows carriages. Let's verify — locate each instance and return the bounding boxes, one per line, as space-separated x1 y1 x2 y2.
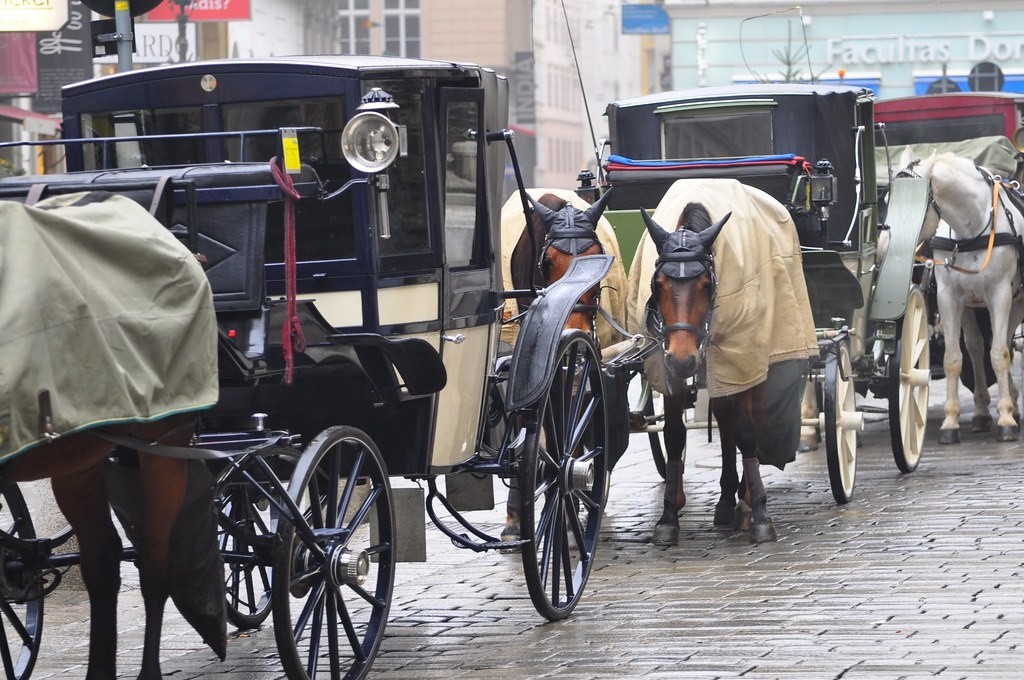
847 86 1023 442
506 75 936 567
1 53 613 680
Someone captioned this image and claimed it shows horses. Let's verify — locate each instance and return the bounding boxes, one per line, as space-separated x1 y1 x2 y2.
874 145 1024 444
626 179 810 550
0 192 202 680
495 187 627 557
